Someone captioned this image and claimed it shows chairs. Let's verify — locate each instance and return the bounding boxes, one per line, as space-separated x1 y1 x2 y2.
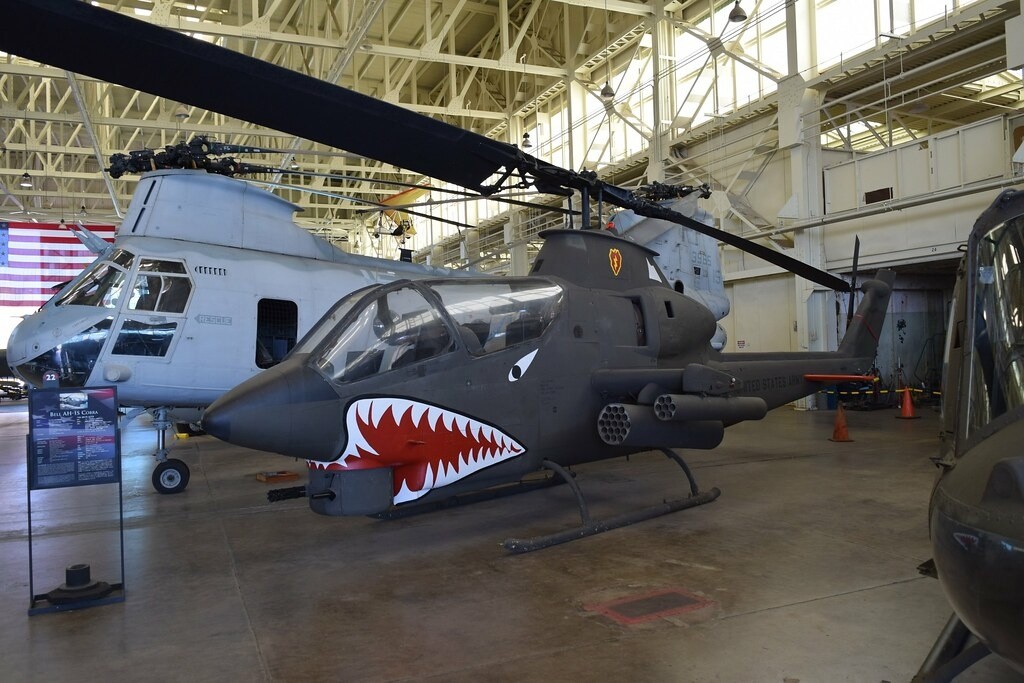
130 295 156 323
390 348 435 372
448 321 491 353
504 319 542 346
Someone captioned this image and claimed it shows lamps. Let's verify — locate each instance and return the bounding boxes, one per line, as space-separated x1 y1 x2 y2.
359 35 373 51
21 106 33 187
79 205 88 216
59 186 67 229
601 0 614 97
522 56 532 147
174 105 189 118
729 0 747 23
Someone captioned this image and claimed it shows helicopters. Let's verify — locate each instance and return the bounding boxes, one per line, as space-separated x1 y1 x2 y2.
0 0 946 552
4 136 737 493
899 178 1022 683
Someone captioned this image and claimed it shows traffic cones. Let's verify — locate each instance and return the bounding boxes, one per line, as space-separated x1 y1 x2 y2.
895 386 923 419
826 400 854 443
